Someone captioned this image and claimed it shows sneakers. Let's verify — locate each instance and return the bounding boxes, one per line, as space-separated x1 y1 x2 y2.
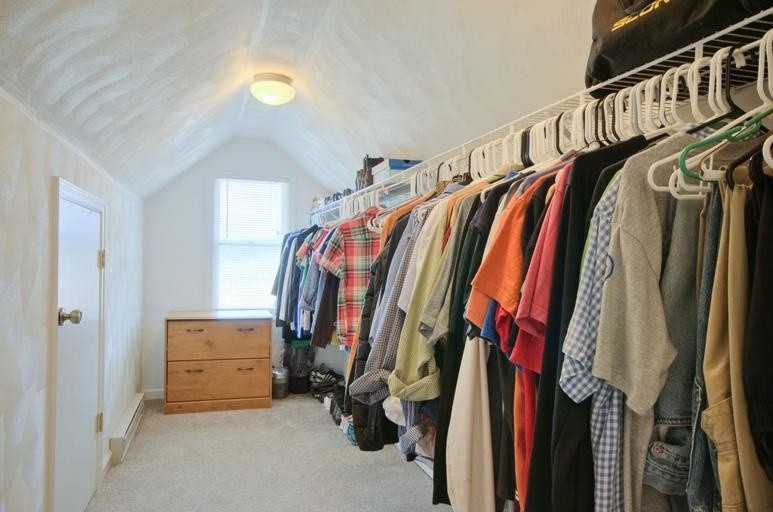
314 386 358 446
315 370 344 382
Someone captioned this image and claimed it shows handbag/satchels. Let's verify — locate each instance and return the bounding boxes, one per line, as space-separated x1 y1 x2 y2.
585 0 773 101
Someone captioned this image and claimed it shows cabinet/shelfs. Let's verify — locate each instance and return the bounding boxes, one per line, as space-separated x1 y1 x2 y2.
164 309 273 415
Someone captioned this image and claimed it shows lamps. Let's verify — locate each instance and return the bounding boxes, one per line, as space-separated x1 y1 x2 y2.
250 73 296 106
